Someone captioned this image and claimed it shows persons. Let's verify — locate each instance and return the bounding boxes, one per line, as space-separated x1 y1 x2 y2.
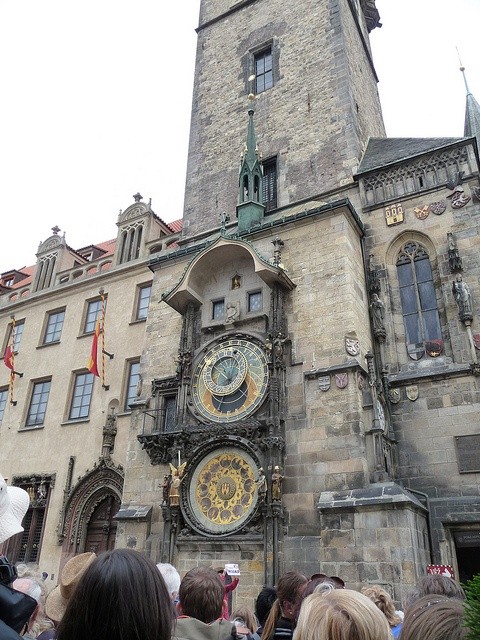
271 465 285 503
452 273 474 315
158 475 168 505
367 293 385 330
169 471 180 505
253 467 268 503
253 570 476 640
47 548 256 640
0 475 47 640
13 577 41 636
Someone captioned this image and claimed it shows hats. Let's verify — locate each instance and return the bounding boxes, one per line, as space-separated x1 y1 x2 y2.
45 552 97 621
0 474 29 544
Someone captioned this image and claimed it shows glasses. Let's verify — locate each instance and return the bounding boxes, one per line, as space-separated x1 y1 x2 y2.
311 573 346 586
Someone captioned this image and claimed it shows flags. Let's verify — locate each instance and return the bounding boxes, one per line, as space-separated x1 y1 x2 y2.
87 312 102 380
4 337 13 369
